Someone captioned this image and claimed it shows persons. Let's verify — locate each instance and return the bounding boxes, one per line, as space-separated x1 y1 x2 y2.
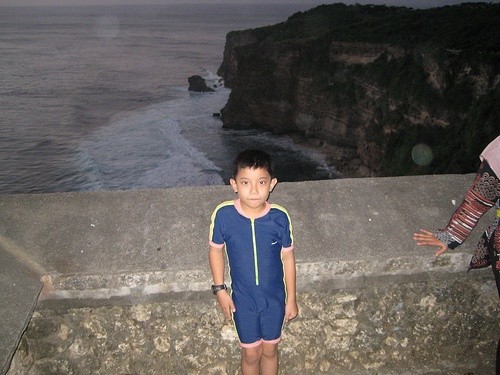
413 135 500 375
207 150 299 375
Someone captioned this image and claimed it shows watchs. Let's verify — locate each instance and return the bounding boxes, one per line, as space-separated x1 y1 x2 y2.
211 284 228 295
